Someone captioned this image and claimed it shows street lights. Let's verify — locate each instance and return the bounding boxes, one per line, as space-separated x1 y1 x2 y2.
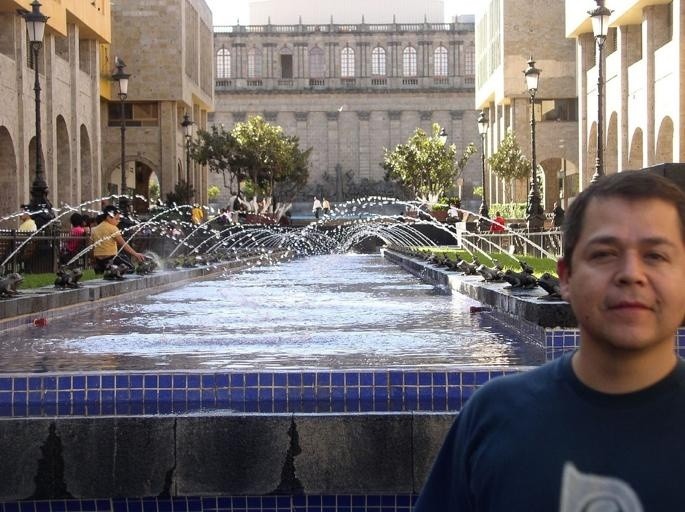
522 55 546 231
476 111 491 225
438 128 448 198
182 111 193 205
111 56 135 222
19 0 59 233
587 0 611 185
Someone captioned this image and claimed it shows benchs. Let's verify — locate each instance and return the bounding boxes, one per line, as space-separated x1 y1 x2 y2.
0 229 40 275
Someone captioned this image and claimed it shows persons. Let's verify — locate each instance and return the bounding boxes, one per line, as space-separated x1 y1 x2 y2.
322 197 330 216
479 215 491 232
410 168 685 510
56 199 246 273
418 200 431 220
551 199 566 232
446 203 460 221
15 203 38 261
491 212 505 231
311 195 323 219
